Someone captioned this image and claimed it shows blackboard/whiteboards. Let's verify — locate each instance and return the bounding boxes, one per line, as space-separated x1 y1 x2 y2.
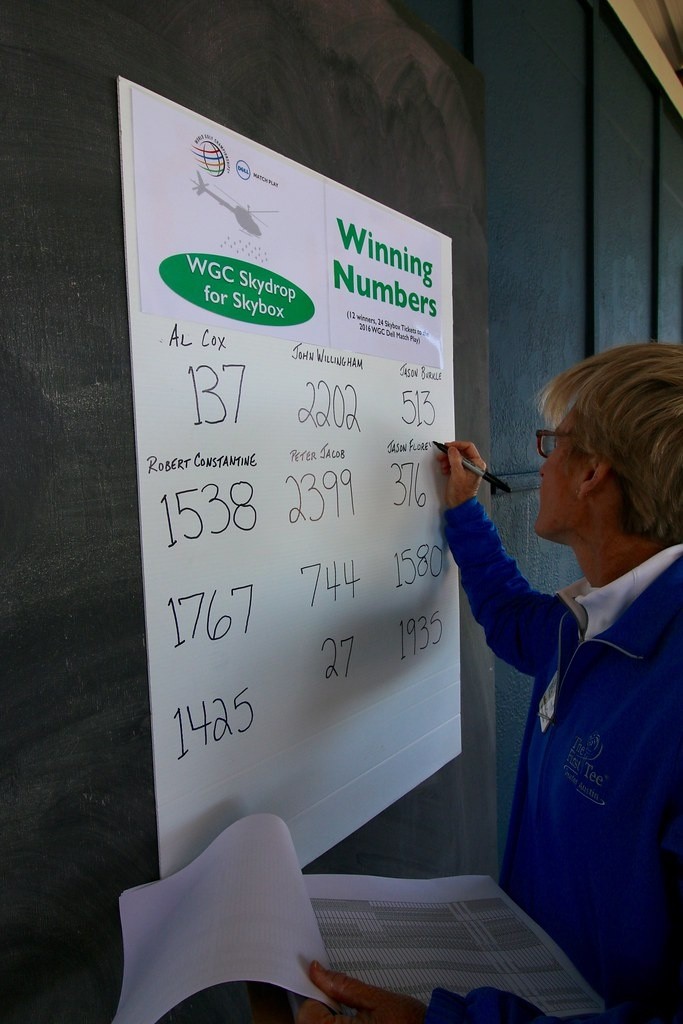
0 0 683 1023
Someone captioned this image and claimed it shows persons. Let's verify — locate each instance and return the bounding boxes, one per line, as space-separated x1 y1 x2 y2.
247 340 682 1024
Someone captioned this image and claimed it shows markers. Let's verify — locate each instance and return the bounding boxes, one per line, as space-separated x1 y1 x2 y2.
432 440 511 494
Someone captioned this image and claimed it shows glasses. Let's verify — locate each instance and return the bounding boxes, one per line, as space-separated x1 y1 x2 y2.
535 430 573 458
